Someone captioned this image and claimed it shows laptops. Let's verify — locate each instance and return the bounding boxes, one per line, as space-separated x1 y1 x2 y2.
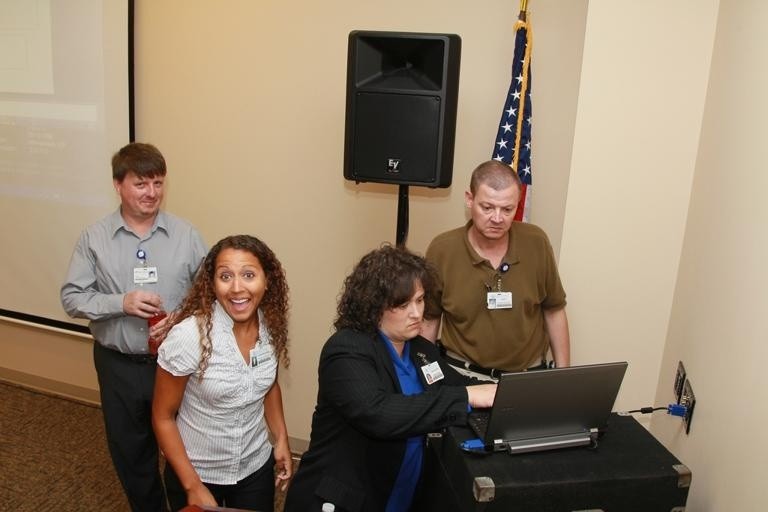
469 361 629 453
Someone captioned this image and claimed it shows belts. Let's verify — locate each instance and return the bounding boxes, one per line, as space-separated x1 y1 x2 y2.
444 356 548 380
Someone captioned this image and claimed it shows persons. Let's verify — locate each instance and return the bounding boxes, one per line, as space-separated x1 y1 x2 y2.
149 235 293 512
426 373 433 383
420 159 571 380
283 242 497 512
59 142 211 512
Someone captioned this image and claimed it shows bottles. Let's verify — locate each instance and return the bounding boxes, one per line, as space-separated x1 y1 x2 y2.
146 304 167 356
320 502 335 512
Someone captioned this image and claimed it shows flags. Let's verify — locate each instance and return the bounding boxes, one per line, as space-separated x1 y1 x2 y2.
491 6 534 224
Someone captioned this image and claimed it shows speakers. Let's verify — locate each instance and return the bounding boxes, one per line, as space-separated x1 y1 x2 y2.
342 30 463 189
